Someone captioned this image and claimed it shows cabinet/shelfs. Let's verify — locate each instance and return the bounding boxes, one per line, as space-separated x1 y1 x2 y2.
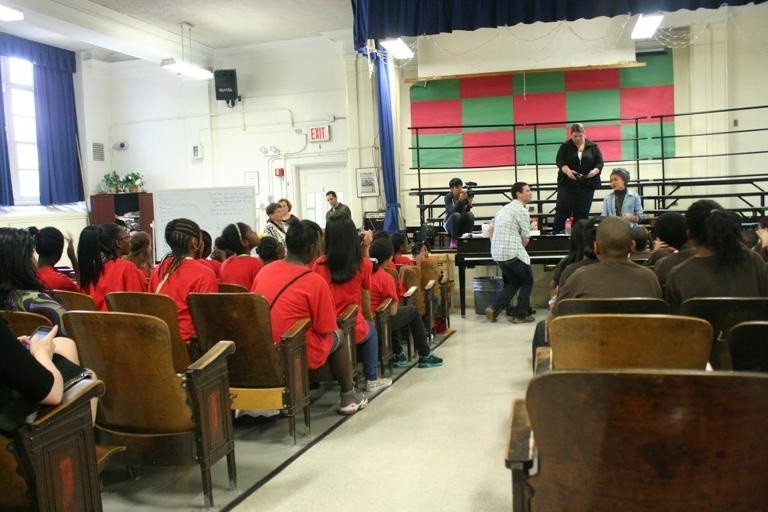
90 192 154 258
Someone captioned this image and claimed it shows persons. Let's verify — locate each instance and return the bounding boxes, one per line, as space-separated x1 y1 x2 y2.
325 227 444 392
196 221 285 294
552 123 603 234
324 191 351 224
484 183 536 323
277 199 300 226
264 204 289 255
601 168 644 228
444 178 474 247
0 219 152 452
253 215 368 415
149 219 217 359
531 212 663 372
648 200 768 313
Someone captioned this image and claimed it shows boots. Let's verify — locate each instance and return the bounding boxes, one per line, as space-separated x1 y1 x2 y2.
338 387 368 415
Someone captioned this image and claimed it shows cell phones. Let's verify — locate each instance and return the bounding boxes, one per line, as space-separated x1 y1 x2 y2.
25 326 52 351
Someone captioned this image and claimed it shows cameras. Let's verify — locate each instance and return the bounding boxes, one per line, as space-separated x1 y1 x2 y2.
464 182 476 198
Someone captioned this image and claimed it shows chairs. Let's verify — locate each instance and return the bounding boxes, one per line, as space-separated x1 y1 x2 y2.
534 313 713 379
719 321 768 371
681 297 768 370
633 259 648 265
533 297 671 366
504 370 766 512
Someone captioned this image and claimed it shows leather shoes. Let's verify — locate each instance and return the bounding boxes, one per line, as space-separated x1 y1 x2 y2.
486 306 497 322
512 316 535 324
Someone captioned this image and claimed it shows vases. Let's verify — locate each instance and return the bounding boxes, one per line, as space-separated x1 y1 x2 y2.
109 185 117 193
128 185 138 192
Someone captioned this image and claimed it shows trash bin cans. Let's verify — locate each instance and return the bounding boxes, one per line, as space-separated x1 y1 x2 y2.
472 276 504 315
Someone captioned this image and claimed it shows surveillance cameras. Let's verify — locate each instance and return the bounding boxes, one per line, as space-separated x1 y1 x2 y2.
119 141 127 148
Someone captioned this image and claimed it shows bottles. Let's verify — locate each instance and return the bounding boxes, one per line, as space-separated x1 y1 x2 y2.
564 218 572 234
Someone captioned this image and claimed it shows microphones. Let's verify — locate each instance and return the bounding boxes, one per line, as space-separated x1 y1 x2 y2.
573 172 584 179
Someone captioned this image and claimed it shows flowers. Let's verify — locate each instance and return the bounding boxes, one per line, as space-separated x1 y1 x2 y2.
120 172 144 190
102 171 122 189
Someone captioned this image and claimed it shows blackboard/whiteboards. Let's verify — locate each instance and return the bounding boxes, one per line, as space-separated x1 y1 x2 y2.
153 184 256 263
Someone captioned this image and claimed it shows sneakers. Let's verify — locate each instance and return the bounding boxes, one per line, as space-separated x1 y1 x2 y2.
365 377 392 392
418 352 443 368
392 352 408 368
450 239 457 249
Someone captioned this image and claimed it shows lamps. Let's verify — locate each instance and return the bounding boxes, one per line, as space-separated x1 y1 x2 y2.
161 22 213 80
0 4 24 23
378 37 414 60
630 13 664 41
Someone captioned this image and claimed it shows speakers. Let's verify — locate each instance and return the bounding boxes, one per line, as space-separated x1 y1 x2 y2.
213 69 237 100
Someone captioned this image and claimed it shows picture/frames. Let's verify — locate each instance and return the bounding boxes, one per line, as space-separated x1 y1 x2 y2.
355 167 380 197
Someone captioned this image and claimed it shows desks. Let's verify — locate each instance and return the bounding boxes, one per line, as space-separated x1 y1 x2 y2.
456 234 652 317
